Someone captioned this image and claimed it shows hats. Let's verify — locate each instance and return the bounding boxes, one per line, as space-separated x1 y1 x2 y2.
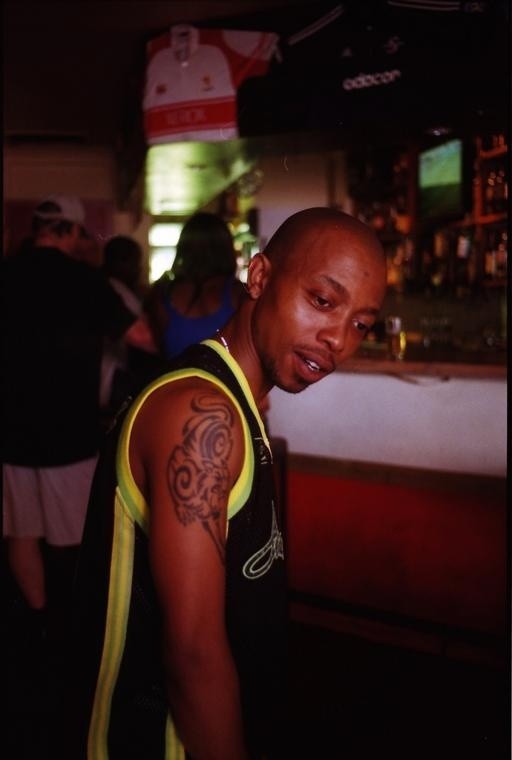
35 187 96 240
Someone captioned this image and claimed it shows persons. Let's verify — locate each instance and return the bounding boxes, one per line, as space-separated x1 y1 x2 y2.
140 209 249 371
0 193 155 610
98 229 145 413
65 206 388 760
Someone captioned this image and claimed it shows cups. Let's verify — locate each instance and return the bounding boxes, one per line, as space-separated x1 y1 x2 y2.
385 317 404 361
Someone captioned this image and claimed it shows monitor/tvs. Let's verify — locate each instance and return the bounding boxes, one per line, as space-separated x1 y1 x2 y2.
415 139 475 225
